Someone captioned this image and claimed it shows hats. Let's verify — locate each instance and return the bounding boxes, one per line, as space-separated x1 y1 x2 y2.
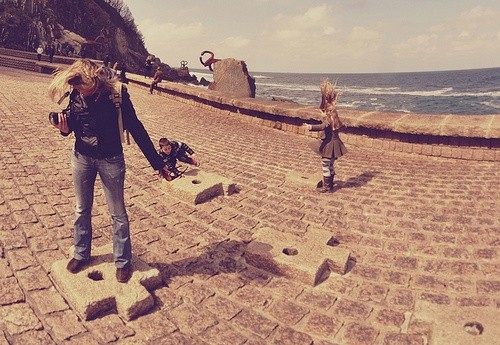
156 67 162 70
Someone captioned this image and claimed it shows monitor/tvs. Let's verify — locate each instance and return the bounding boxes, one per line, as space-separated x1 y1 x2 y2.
49 108 72 126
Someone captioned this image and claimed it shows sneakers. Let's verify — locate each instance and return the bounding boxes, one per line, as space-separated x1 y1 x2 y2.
115 266 132 282
66 258 86 274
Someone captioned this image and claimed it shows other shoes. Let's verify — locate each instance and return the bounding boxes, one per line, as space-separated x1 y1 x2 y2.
193 160 200 167
148 91 153 94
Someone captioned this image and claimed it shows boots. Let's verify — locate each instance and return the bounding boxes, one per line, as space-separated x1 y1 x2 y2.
316 174 335 193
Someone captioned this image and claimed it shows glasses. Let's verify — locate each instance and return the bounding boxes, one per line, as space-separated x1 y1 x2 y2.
165 165 189 181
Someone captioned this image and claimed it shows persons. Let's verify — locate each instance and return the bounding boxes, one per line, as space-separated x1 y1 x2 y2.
158 138 200 177
37 42 55 62
303 81 348 193
103 55 164 94
47 60 172 282
